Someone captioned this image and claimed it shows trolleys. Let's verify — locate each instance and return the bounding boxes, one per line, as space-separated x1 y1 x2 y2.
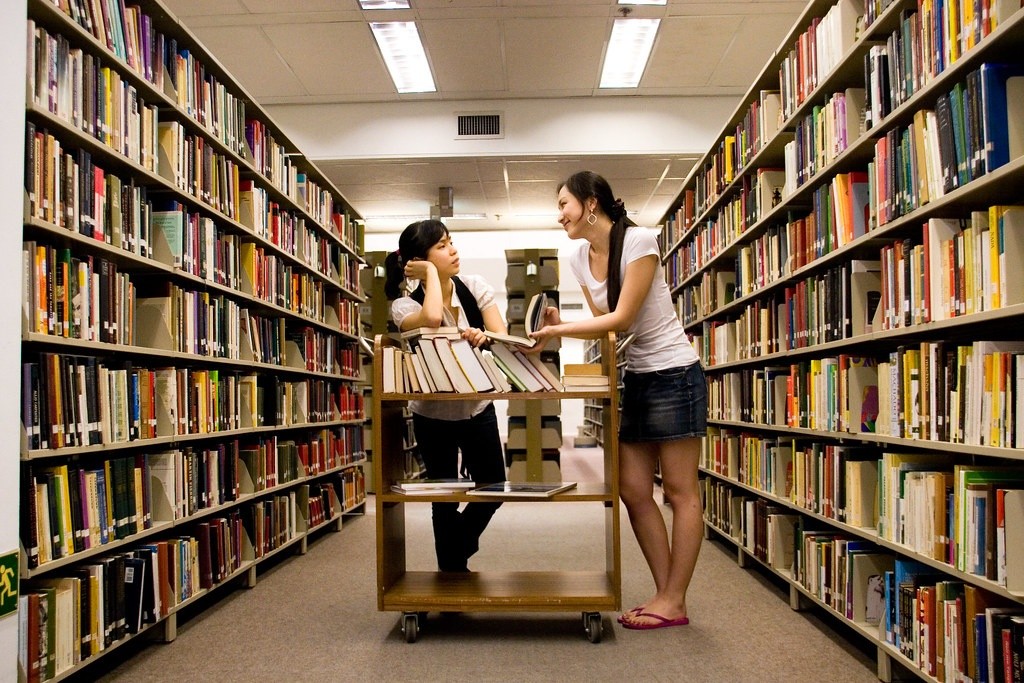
369 325 623 645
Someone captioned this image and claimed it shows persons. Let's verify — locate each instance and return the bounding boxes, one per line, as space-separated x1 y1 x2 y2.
385 220 515 572
513 170 707 630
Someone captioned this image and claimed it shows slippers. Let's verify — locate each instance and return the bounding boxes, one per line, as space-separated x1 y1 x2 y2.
617 605 645 622
623 609 689 630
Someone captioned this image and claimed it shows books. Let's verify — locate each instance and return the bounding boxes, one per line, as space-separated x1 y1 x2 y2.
21 0 1024 683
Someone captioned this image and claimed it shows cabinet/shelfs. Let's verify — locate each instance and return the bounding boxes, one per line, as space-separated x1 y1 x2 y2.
404 415 426 480
374 330 622 645
17 0 369 683
504 249 563 491
583 334 626 447
655 0 1024 683
